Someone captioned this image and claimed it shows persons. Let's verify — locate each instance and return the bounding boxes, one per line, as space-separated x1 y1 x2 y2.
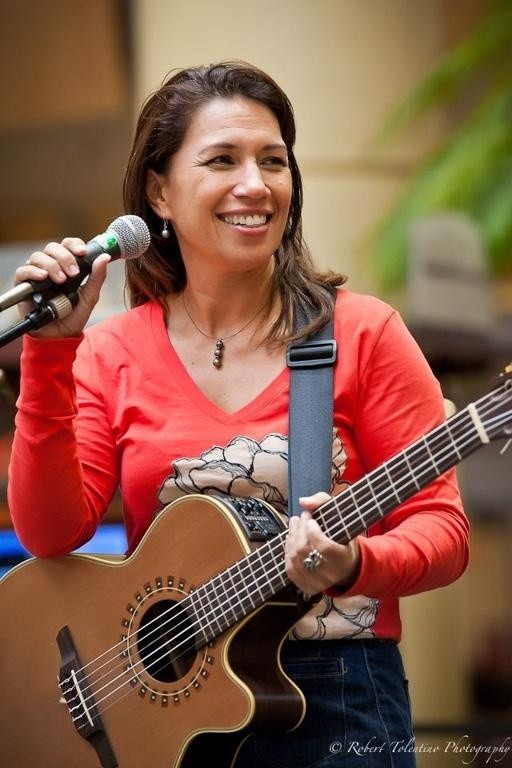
5 60 471 767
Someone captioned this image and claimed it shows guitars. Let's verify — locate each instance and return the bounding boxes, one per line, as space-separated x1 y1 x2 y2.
1 368 511 766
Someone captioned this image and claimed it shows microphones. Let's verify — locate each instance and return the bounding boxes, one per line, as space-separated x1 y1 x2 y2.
27 214 152 292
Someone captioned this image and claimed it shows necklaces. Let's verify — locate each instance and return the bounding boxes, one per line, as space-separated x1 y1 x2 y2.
179 286 271 369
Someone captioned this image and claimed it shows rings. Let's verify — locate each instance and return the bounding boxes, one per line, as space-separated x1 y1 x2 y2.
301 549 325 572
